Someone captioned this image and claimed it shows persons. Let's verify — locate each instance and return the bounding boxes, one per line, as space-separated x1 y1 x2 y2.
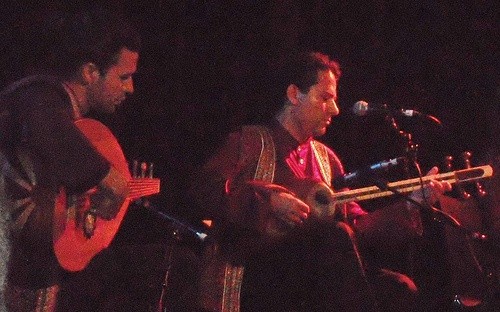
184 11 452 312
0 11 140 312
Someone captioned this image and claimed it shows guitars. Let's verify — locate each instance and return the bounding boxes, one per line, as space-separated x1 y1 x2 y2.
50 118 160 272
258 150 492 247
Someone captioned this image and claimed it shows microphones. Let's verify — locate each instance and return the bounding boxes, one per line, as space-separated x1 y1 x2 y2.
352 100 421 119
332 157 405 190
148 207 208 241
